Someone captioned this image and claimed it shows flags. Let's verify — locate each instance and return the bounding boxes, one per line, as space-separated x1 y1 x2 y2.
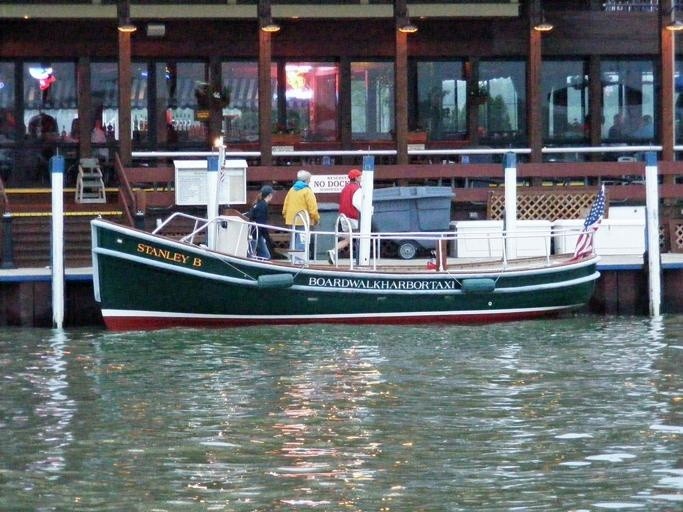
562 183 606 262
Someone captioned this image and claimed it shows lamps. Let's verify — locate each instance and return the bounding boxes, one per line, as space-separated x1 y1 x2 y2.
662 4 683 31
259 8 282 33
397 6 419 34
532 8 555 32
116 7 138 33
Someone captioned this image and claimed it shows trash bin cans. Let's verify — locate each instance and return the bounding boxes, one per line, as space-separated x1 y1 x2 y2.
308 202 341 260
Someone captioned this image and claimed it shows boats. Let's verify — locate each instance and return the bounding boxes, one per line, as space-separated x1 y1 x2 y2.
90 209 602 331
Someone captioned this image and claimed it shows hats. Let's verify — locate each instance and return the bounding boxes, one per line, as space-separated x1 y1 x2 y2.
348 169 362 179
296 170 311 181
260 185 277 194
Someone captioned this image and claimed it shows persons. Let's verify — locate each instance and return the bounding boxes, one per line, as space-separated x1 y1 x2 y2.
281 169 320 263
3 109 24 137
606 113 655 139
326 168 374 266
90 117 108 145
245 186 272 259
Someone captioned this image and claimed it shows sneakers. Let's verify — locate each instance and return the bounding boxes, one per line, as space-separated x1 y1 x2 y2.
327 249 335 265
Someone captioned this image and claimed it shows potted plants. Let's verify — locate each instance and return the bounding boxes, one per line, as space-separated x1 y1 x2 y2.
466 78 490 106
193 77 232 111
